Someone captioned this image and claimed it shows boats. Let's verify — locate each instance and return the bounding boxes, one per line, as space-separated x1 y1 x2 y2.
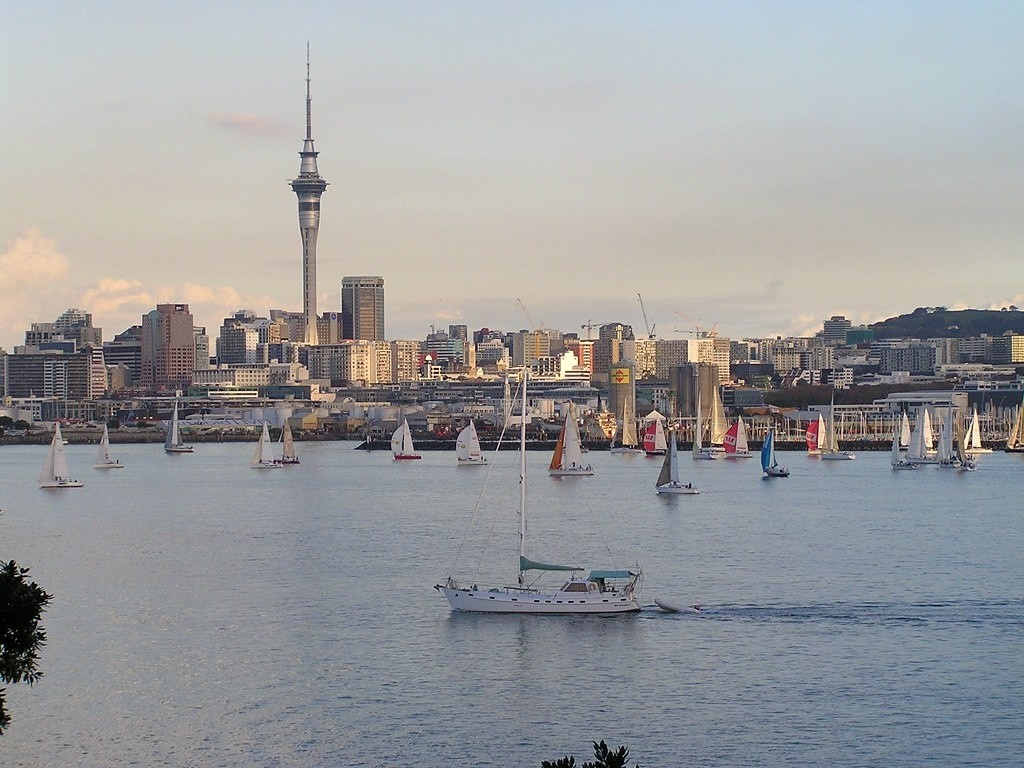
655 597 702 614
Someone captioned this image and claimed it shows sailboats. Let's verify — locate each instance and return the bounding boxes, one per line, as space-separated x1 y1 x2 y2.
610 397 644 453
760 428 791 477
642 415 668 455
690 385 1024 473
250 422 285 469
454 419 490 465
94 420 124 468
431 365 645 614
560 401 588 455
162 398 194 453
273 418 301 464
653 427 700 494
37 421 87 488
547 410 596 476
390 418 422 459
